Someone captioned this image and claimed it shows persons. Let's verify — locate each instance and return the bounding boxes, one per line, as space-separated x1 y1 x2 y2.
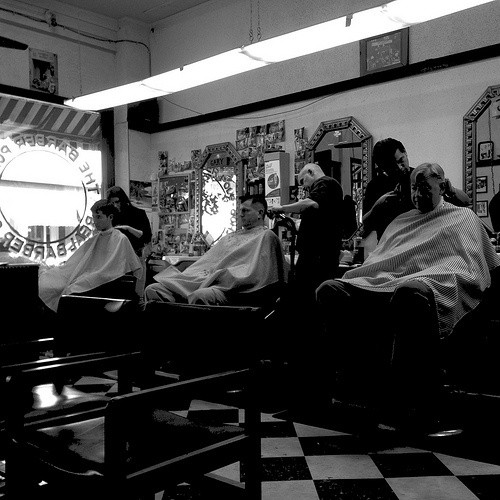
106 188 153 302
266 163 345 381
362 137 470 257
145 192 285 310
314 162 499 404
46 184 144 362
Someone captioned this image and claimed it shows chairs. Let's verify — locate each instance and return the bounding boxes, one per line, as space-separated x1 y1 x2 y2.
0 274 262 500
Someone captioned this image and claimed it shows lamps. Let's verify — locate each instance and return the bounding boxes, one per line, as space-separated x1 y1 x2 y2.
62 0 500 111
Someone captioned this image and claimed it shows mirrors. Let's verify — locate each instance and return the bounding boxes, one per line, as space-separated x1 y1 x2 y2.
193 142 244 246
462 84 500 238
305 116 372 248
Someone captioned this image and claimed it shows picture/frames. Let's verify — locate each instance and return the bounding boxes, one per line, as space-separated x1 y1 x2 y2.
359 28 409 78
